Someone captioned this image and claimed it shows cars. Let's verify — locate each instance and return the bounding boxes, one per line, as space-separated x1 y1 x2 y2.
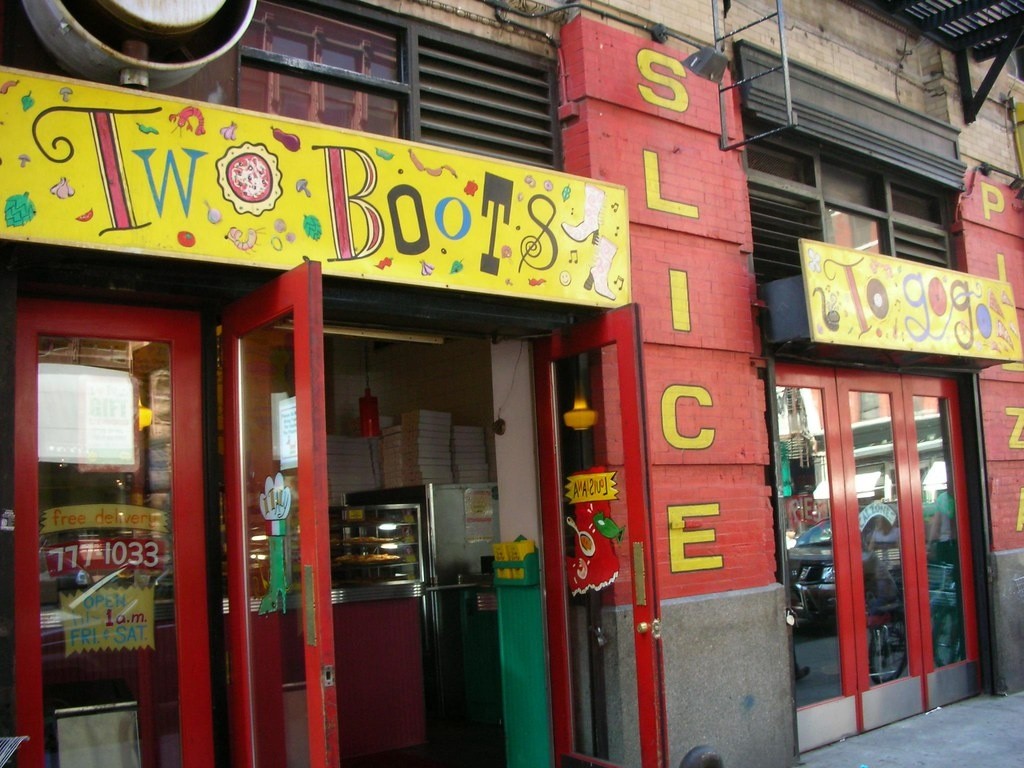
786 518 837 633
37 564 174 703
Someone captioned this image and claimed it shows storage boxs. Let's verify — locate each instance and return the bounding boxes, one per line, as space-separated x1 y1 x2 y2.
377 409 489 489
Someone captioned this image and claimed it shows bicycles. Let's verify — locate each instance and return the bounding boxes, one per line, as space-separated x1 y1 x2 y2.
870 587 962 684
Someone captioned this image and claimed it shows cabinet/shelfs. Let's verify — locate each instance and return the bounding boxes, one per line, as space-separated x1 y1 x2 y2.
60 502 426 768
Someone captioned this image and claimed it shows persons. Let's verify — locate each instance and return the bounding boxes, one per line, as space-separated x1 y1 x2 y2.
927 482 955 563
787 608 809 680
867 505 900 552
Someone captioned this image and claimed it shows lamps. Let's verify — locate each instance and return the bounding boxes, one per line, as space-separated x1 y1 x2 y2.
137 397 152 432
563 355 596 431
653 23 728 86
359 337 381 438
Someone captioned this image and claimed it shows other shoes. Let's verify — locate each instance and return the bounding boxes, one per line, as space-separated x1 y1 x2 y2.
796 666 809 679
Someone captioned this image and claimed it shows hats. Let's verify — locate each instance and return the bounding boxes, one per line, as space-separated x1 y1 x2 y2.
56 571 94 591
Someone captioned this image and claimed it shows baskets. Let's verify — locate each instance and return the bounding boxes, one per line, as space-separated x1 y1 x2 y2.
929 564 958 606
